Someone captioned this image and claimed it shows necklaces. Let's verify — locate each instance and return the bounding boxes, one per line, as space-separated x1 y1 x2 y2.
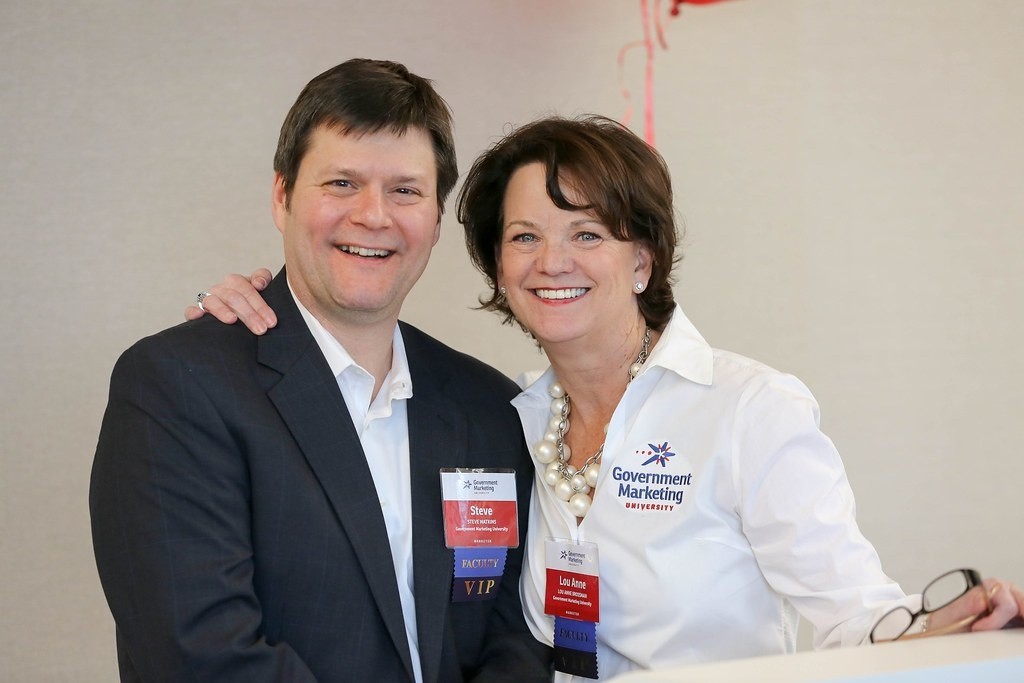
533 326 652 516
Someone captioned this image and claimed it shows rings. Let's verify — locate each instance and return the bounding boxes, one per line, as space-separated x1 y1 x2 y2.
196 290 212 312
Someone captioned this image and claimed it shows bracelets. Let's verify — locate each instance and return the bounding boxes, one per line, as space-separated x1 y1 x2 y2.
920 615 928 632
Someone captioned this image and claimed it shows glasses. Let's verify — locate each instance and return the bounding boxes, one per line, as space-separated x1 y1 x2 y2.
869 569 992 644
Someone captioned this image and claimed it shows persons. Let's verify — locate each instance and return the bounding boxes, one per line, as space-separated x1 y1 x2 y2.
184 117 1024 683
89 58 554 683
